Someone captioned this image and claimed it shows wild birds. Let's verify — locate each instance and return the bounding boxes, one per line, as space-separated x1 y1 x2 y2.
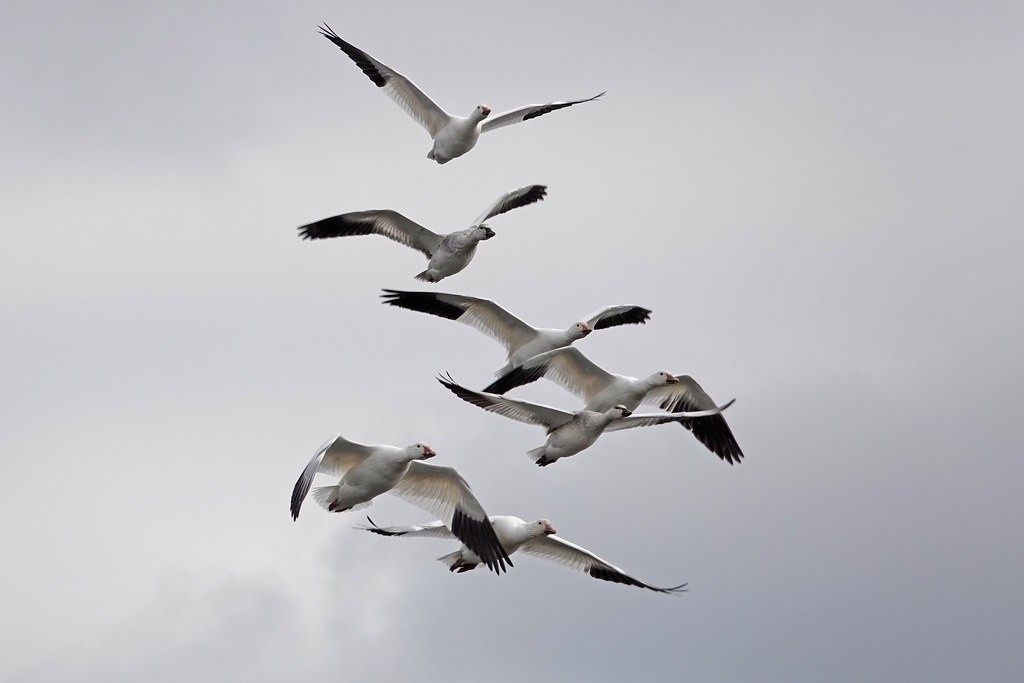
289 185 745 595
316 21 607 165
